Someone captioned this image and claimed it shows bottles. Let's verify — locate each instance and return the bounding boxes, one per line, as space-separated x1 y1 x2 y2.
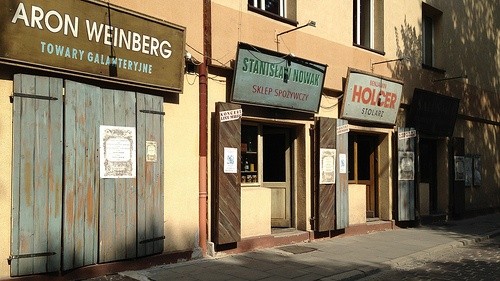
247 137 253 152
241 156 249 171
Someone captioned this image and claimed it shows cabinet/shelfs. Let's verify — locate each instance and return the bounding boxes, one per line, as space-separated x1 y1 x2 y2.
241 152 257 173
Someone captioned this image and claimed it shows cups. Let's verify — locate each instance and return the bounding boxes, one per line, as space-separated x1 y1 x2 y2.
242 175 257 182
250 164 254 171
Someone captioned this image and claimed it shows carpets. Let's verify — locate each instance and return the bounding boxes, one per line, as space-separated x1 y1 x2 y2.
278 245 318 255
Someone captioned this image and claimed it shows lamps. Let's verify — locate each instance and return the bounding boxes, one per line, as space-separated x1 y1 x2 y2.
371 54 410 70
276 20 317 43
431 73 472 87
